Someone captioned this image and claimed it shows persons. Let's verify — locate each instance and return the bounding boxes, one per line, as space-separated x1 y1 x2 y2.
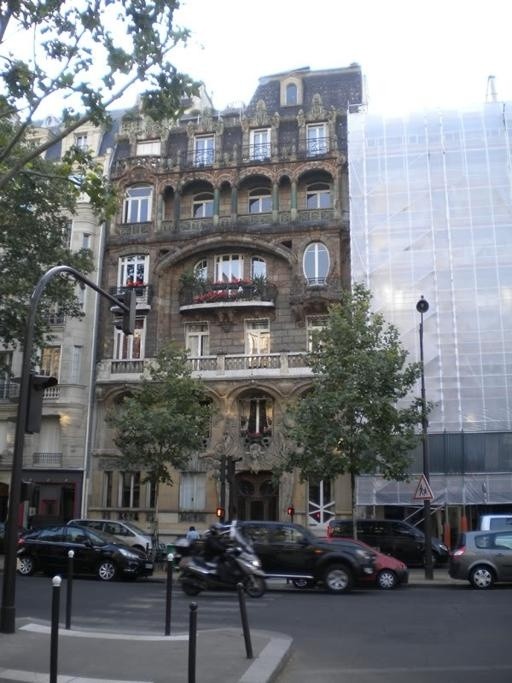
185 526 199 542
204 522 242 582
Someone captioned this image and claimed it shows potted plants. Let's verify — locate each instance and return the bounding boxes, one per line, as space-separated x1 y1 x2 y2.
178 268 196 286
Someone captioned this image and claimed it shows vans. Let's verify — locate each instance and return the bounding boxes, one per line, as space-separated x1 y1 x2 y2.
476 514 512 549
327 518 452 570
64 518 155 557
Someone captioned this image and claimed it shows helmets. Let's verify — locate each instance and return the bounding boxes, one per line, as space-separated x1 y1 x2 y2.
210 523 224 536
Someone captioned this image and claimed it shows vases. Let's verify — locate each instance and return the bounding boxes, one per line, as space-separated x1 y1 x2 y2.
124 287 142 293
211 285 253 290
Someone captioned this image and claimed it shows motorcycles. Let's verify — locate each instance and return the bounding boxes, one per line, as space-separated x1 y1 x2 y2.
176 545 267 598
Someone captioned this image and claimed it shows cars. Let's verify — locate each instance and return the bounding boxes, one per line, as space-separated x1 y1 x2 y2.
293 536 409 590
448 528 512 591
15 524 154 582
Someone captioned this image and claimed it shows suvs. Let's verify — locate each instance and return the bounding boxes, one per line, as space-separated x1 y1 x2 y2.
192 521 378 592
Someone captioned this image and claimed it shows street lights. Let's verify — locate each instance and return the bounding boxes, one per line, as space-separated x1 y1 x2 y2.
414 294 434 581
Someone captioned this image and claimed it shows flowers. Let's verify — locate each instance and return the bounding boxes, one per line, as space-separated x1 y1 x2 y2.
192 290 238 303
127 280 142 286
212 278 254 285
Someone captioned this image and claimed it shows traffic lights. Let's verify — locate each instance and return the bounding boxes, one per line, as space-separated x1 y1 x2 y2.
287 507 294 515
110 289 137 335
215 507 223 517
27 374 58 435
226 454 243 481
